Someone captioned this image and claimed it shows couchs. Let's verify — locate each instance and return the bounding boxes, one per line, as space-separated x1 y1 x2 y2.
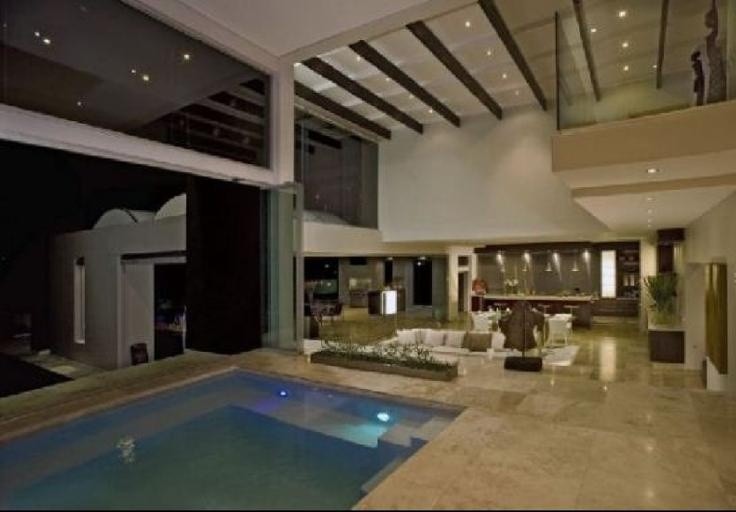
379 327 504 365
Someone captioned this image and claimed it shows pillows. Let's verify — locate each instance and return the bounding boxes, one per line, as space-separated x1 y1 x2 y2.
448 331 466 349
422 329 444 346
466 330 491 351
396 330 415 348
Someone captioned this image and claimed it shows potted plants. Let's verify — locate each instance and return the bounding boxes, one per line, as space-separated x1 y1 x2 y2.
309 330 459 382
642 272 683 328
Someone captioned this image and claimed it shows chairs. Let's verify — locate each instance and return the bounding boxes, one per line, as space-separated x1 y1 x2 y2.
323 302 345 325
467 304 573 348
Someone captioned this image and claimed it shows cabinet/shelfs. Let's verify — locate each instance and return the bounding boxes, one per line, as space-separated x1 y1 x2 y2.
647 309 685 363
